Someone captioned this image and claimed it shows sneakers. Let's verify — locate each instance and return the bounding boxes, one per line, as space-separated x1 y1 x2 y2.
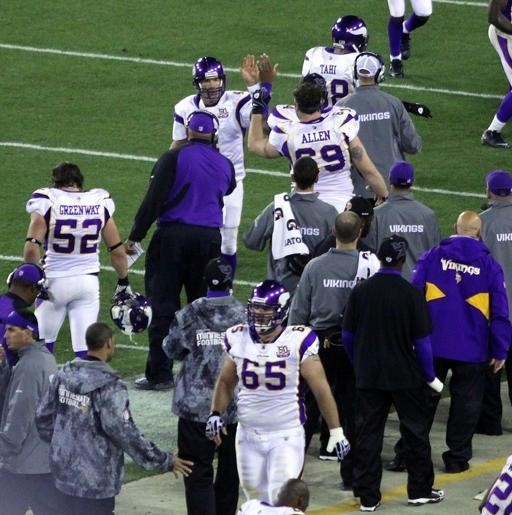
385 460 408 472
388 33 410 77
481 131 509 148
135 376 174 389
408 488 444 505
360 502 380 512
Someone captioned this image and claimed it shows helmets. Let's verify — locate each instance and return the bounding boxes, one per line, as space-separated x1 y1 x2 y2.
110 292 153 334
192 56 225 99
332 15 385 82
247 280 291 334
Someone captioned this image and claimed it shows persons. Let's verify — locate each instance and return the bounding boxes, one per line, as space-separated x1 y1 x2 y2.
1 162 193 514
126 1 512 514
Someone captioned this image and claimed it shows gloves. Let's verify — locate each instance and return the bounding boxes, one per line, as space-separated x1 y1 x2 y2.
326 427 351 460
205 411 227 440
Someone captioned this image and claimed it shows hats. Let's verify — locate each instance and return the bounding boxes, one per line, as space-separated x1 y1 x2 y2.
378 235 408 263
14 264 42 281
389 160 413 185
345 196 374 216
206 258 233 285
0 311 37 332
189 113 214 133
487 170 512 196
